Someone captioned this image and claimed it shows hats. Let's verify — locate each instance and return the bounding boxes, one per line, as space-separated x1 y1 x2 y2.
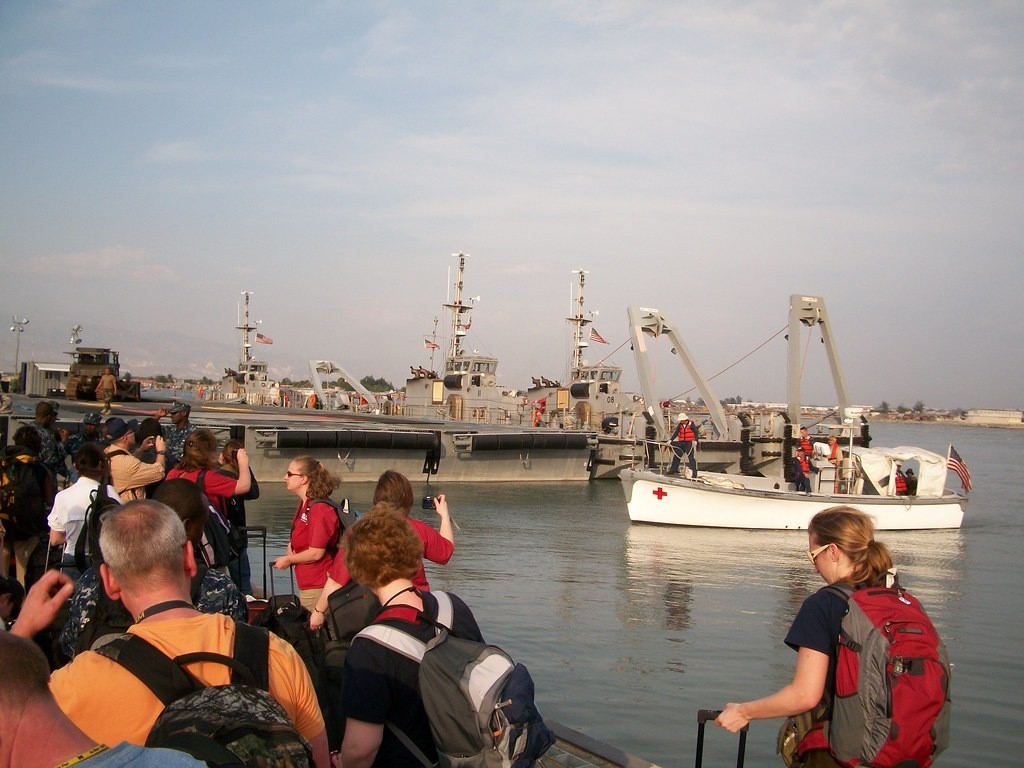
168 402 190 413
796 447 805 452
905 469 914 476
83 412 102 426
108 418 138 439
49 401 60 414
677 413 689 421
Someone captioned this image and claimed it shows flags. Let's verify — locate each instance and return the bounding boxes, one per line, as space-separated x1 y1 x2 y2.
946 445 973 494
424 338 440 351
590 327 610 345
255 332 273 344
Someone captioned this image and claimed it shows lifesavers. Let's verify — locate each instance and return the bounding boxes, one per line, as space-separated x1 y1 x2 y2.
283 394 289 405
853 478 865 495
198 388 203 397
530 410 540 425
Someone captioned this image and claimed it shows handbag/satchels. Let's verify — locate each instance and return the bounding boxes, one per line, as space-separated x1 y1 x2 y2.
773 707 827 768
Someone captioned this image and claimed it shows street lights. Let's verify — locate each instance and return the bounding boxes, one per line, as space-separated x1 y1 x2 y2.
10 314 29 376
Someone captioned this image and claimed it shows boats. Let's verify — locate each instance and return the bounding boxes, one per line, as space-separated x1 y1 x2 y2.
618 409 968 530
584 293 873 480
198 251 634 431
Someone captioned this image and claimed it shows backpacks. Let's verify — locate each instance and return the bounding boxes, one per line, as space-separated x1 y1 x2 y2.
0 461 39 540
828 587 957 768
306 497 363 531
94 623 321 768
325 580 382 643
75 483 121 574
101 450 128 485
352 589 558 768
75 563 211 653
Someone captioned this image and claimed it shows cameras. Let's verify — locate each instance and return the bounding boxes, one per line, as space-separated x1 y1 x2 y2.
150 438 157 444
422 495 440 510
231 449 239 460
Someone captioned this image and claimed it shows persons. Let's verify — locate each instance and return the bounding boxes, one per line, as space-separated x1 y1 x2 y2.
895 461 918 496
9 499 332 768
273 454 340 614
0 629 210 768
94 367 117 416
55 478 249 661
332 501 486 768
713 505 950 768
0 576 25 631
665 413 699 478
310 470 455 631
0 400 260 598
823 435 844 495
784 426 824 497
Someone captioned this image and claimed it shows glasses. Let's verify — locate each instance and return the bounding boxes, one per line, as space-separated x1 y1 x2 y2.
287 471 303 477
124 429 136 438
806 544 830 565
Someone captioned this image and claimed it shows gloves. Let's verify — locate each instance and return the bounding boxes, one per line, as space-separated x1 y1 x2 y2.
692 441 697 446
665 440 671 444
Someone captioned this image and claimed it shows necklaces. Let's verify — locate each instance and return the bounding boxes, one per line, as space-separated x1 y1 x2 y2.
56 743 108 768
382 586 423 607
135 600 199 625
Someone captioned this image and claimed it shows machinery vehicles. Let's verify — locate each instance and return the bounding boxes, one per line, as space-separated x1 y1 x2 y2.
63 346 141 403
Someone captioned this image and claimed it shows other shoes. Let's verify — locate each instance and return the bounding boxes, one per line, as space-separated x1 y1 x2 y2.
100 409 112 415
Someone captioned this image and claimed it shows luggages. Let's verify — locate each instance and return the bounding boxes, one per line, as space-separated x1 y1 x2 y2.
237 525 268 623
266 561 302 613
